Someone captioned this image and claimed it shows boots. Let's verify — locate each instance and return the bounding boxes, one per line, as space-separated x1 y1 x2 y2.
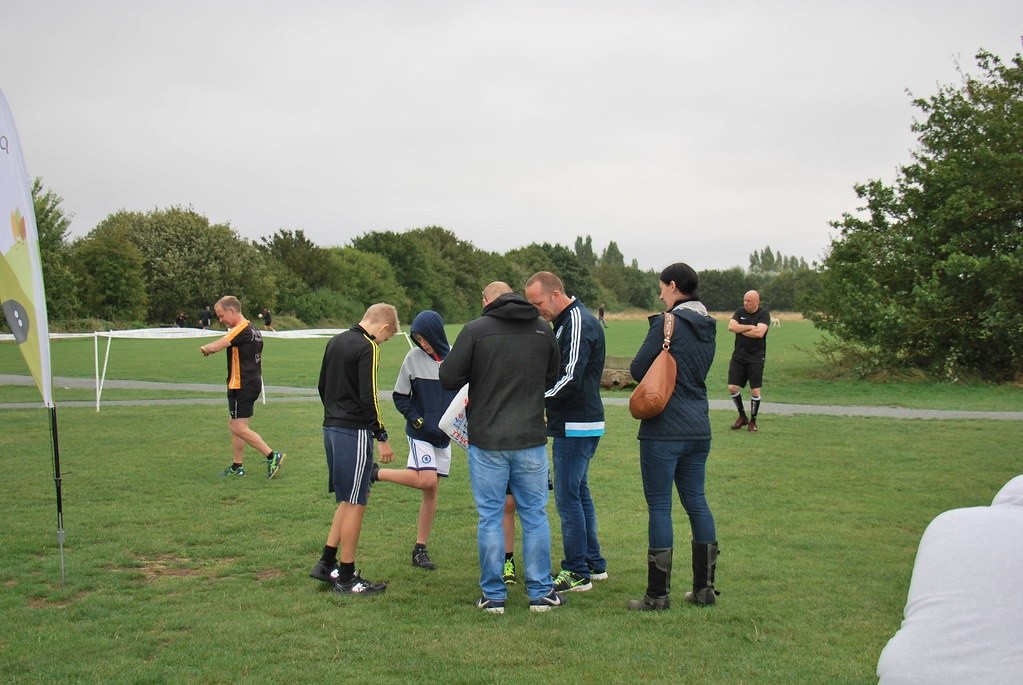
684 538 720 606
628 546 673 610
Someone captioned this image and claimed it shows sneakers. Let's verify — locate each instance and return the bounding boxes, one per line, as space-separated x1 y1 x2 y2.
552 569 593 593
502 557 516 584
475 594 505 614
334 569 387 594
267 450 286 481
589 567 608 581
413 547 436 569
530 588 567 612
731 416 749 429
369 462 378 492
309 555 341 584
218 466 245 477
748 421 757 432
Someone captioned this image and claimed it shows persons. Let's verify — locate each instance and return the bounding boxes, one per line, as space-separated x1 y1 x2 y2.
199 296 287 480
877 472 1023 685
441 282 567 613
524 271 608 594
369 310 453 571
728 289 770 431
627 263 720 611
598 303 608 328
309 303 400 595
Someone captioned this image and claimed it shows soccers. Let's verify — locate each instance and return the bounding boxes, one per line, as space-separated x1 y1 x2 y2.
258 313 263 319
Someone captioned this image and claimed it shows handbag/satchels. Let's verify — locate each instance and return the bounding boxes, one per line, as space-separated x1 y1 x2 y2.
628 312 678 419
438 380 468 452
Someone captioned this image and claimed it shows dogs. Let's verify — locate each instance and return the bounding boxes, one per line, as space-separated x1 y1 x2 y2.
771 318 780 328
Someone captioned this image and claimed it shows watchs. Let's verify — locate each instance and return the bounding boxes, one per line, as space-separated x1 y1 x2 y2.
377 433 388 442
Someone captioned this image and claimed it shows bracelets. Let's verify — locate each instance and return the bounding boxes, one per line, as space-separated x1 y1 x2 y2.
413 417 424 429
